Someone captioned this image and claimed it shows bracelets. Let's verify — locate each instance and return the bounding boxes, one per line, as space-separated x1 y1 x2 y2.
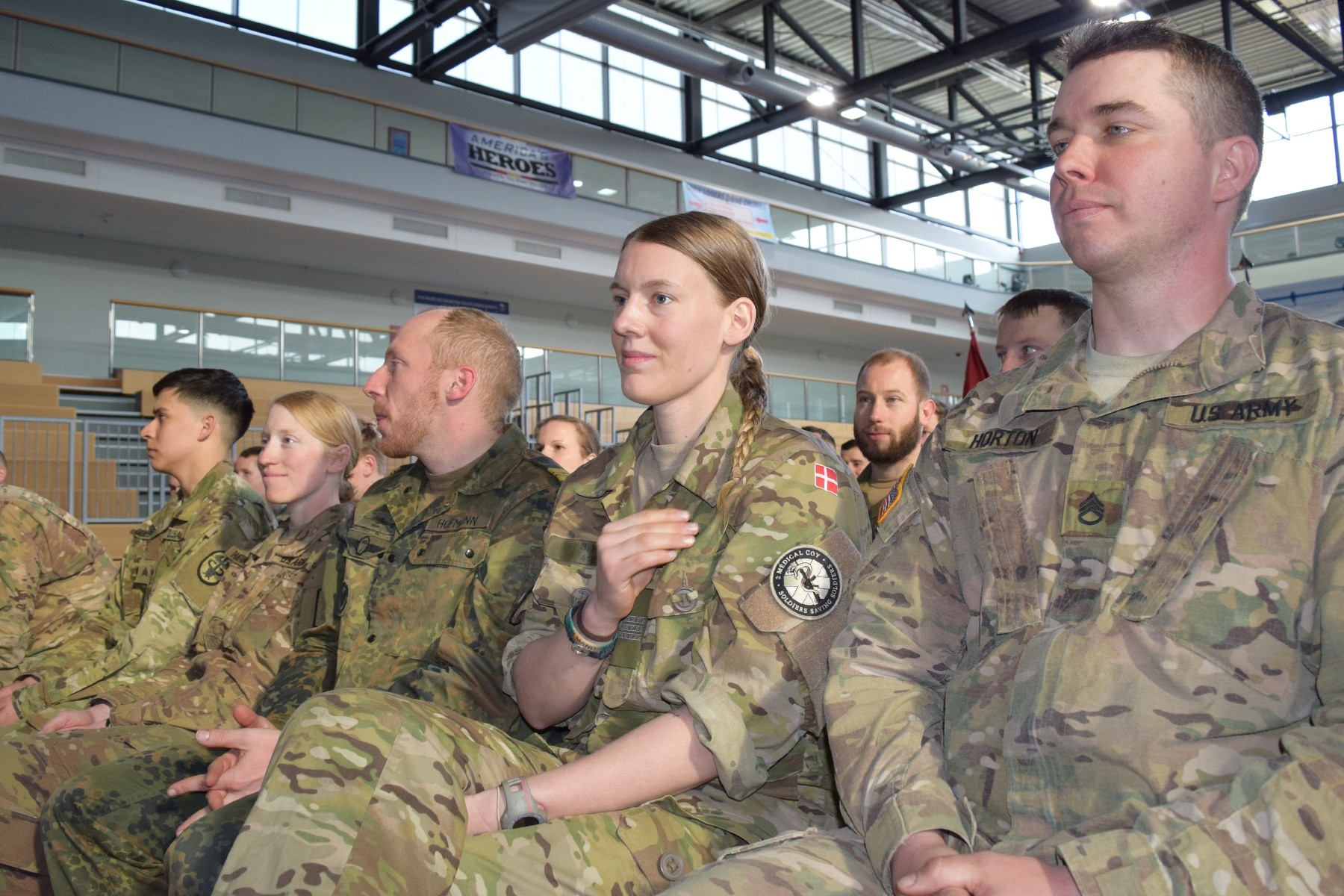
564 594 618 661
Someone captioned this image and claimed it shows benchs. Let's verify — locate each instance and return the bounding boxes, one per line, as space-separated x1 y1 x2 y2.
0 360 855 569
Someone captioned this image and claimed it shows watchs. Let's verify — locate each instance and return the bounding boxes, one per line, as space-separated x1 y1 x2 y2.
500 777 547 829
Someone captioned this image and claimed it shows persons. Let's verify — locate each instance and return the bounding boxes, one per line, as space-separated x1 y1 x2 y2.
534 415 600 474
0 367 387 896
210 212 876 896
36 307 571 896
656 24 1344 896
796 288 1094 541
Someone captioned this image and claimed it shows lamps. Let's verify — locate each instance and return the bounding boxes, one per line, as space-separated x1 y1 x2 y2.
838 105 866 120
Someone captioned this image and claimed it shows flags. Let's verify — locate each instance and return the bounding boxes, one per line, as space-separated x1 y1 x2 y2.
963 333 990 399
